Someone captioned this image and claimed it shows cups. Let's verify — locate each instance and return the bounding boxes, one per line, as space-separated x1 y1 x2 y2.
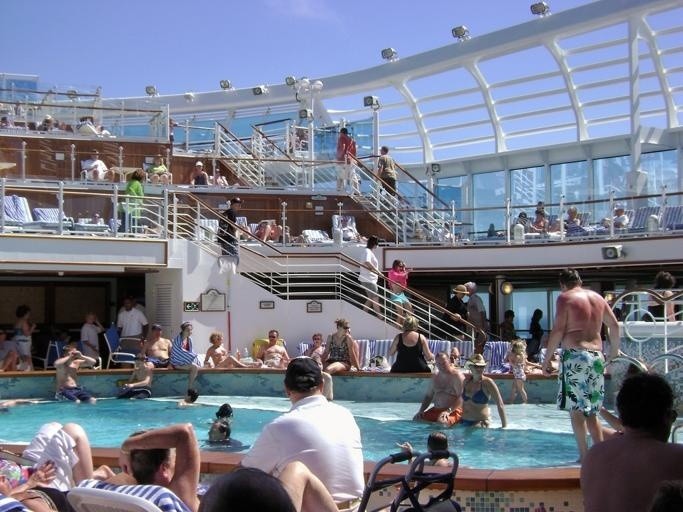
370 363 376 373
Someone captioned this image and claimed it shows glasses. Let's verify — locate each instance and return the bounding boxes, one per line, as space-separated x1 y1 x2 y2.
268 336 275 338
397 265 402 267
667 410 677 420
471 365 484 368
340 326 349 329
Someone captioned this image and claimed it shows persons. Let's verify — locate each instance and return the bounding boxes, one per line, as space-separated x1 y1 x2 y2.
0 116 242 190
386 270 683 511
0 298 367 510
86 169 148 235
216 195 305 265
486 200 628 238
336 216 414 329
288 125 397 207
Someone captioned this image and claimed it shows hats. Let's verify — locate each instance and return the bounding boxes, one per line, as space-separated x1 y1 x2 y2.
469 354 487 366
230 197 240 204
258 219 270 225
44 114 51 119
195 161 202 166
284 357 321 392
452 285 469 293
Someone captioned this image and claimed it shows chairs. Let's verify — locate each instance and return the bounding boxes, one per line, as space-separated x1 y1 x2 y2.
252 339 285 360
0 196 109 239
76 121 97 134
208 174 228 186
476 206 683 241
302 215 355 242
32 340 102 370
194 218 220 243
297 340 515 374
78 217 104 239
67 486 162 512
540 348 562 363
234 217 258 240
143 155 173 185
79 159 115 181
103 326 143 368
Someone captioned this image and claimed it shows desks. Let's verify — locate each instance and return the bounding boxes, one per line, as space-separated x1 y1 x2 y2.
110 167 139 182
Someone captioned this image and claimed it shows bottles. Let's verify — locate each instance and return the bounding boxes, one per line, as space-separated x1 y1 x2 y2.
235 349 241 361
243 347 248 357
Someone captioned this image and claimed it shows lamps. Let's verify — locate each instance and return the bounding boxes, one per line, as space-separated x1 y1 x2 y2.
603 245 626 260
145 86 157 97
253 84 270 97
219 79 231 89
364 95 381 114
530 0 552 16
451 25 473 41
431 162 442 174
381 47 401 62
183 92 196 103
67 89 81 103
285 75 323 118
